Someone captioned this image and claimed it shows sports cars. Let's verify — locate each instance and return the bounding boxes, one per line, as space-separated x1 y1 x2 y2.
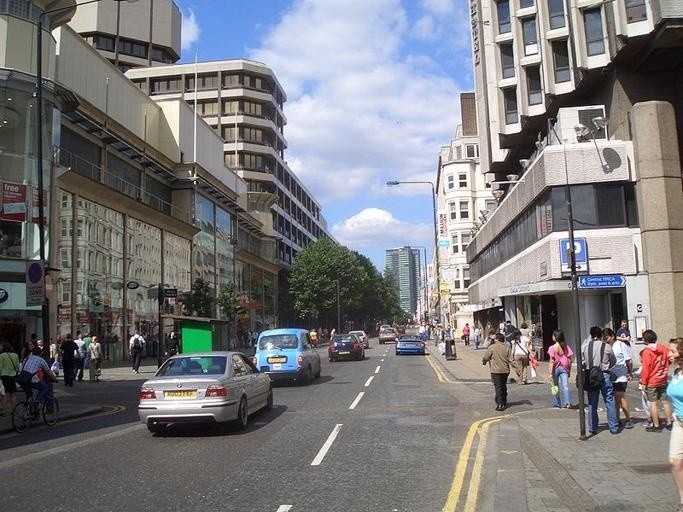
394 334 425 356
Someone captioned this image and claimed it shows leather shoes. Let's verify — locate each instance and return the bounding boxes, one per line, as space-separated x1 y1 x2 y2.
496 404 505 410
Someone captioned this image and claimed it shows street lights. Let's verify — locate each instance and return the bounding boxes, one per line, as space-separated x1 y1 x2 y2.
331 279 341 335
402 243 428 318
385 180 441 323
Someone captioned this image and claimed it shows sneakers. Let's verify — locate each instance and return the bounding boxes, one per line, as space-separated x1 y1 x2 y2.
665 424 672 430
623 421 632 429
646 425 662 433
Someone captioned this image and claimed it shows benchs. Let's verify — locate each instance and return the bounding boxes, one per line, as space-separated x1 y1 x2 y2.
169 365 223 374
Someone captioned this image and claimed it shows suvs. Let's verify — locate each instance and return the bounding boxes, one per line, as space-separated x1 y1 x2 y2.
252 327 321 385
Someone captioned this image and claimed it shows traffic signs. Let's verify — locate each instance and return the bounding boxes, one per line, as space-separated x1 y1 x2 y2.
147 287 158 299
578 274 626 289
163 288 178 298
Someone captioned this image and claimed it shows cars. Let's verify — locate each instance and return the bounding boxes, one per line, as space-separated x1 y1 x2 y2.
137 351 274 431
397 326 405 334
379 325 390 332
327 334 365 361
348 330 369 349
378 328 399 343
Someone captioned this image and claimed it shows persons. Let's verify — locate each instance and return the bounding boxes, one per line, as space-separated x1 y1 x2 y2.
547 330 574 408
0 331 102 418
482 333 510 410
308 326 336 345
666 336 682 510
426 323 452 354
582 322 672 434
129 330 145 374
503 322 531 384
230 328 259 350
165 330 179 359
473 322 481 349
462 323 471 345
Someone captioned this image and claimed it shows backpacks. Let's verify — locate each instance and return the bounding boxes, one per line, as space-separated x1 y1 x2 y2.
134 338 141 350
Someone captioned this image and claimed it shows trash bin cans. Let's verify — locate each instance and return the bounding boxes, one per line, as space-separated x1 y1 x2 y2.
446 339 457 360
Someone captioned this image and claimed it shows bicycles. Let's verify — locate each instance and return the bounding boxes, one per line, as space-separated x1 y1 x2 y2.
11 377 59 435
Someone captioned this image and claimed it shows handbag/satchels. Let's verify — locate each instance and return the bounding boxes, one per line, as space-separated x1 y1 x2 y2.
551 385 560 396
16 369 33 386
583 366 605 392
